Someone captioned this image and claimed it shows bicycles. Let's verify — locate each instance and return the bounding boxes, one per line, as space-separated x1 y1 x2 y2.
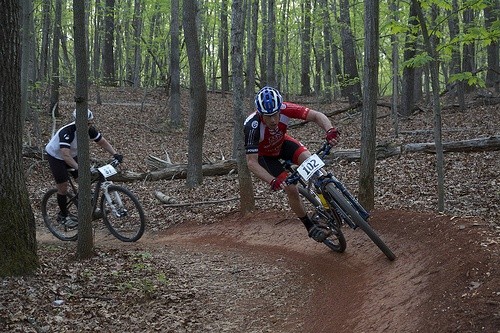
40 158 146 242
270 127 397 263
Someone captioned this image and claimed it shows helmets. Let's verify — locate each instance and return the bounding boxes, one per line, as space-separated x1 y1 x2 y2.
255 86 284 117
71 108 94 122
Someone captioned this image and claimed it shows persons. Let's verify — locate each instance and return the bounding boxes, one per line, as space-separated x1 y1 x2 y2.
45 108 123 228
242 87 342 243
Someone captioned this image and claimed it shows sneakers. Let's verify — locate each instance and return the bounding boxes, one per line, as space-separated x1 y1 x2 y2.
56 215 77 229
308 224 327 243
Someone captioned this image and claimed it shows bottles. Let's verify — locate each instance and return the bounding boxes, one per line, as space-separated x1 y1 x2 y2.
314 185 330 210
